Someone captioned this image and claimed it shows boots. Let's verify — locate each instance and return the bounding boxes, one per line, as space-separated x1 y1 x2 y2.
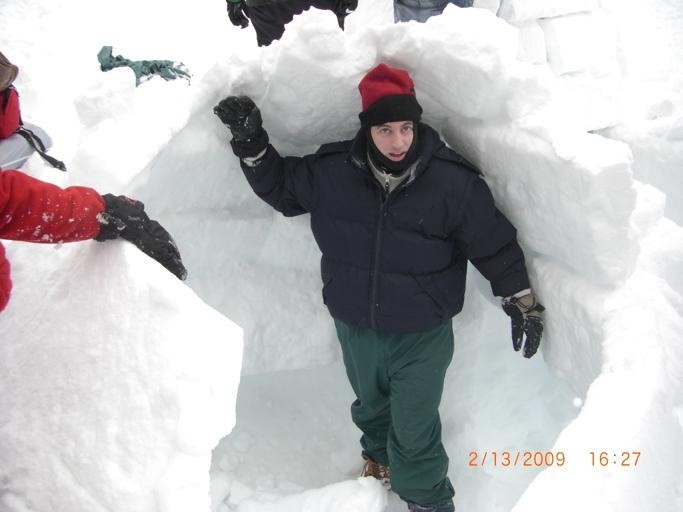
356 457 391 492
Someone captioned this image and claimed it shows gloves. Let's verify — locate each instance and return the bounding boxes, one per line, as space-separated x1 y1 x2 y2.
96 194 189 280
501 288 545 359
226 0 250 28
211 95 270 160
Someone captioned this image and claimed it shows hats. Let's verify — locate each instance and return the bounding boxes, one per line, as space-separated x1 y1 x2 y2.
358 62 422 125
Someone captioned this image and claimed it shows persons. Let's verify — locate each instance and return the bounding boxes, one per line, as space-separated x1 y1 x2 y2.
213 64 545 512
0 52 188 313
